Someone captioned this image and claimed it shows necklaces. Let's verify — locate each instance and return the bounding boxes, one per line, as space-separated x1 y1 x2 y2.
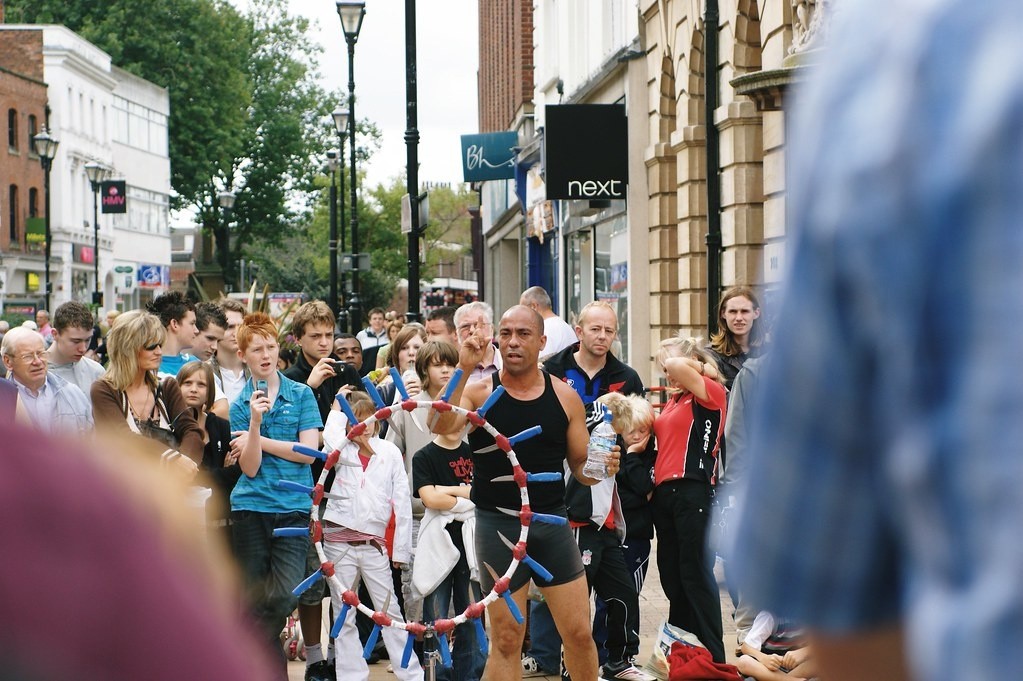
128 384 150 423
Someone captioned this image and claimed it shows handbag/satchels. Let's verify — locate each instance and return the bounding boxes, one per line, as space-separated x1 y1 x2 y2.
145 380 181 450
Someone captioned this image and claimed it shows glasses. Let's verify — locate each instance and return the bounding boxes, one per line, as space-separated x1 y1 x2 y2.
144 344 161 350
5 352 51 363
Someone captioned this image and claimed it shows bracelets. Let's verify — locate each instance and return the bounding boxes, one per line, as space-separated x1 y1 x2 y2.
701 362 704 375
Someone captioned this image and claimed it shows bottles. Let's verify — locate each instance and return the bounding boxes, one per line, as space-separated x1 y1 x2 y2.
583 409 616 481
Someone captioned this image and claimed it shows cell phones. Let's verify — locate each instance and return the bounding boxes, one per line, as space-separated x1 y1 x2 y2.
255 379 268 403
327 360 346 372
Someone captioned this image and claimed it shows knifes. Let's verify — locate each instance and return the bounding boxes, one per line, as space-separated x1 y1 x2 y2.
272 525 349 538
435 382 567 667
428 367 463 435
362 590 392 659
336 394 375 457
329 568 362 638
399 591 424 670
292 446 364 468
292 548 350 598
360 376 406 438
274 479 349 504
388 367 423 433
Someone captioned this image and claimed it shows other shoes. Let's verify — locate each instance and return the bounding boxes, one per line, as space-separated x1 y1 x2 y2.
305 660 336 681
597 662 657 681
522 656 551 677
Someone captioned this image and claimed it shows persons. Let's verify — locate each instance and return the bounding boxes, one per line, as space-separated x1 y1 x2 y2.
520 285 658 681
0 289 505 681
706 285 819 680
704 0 1023 681
428 304 622 681
652 336 727 664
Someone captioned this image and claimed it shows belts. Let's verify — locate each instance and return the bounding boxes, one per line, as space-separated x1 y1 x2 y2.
347 539 383 556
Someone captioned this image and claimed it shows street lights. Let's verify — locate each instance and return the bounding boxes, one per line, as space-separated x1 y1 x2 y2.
33 123 61 312
336 0 366 338
325 150 339 323
331 98 350 333
85 156 107 320
218 184 237 293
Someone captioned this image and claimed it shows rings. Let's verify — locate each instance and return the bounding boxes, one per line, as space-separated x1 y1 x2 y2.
618 458 620 466
